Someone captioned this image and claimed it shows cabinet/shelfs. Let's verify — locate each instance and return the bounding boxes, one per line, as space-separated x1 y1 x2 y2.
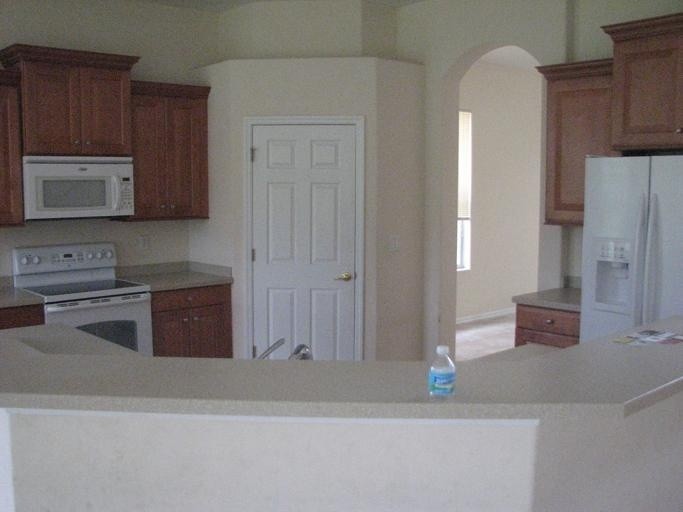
600 11 683 152
0 44 142 156
532 58 624 229
151 283 232 359
514 304 581 348
110 80 211 222
0 68 24 227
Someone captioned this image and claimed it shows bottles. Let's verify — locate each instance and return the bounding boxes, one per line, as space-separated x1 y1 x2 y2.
427 345 457 403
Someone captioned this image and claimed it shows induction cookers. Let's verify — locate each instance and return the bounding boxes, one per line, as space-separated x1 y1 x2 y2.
26 279 151 303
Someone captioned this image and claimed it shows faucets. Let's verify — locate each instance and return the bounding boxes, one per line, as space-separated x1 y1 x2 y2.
287 343 313 358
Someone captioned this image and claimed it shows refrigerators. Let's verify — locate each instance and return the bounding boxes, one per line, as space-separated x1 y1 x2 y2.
579 152 683 343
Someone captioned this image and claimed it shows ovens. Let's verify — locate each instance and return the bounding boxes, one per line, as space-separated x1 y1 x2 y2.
44 292 154 358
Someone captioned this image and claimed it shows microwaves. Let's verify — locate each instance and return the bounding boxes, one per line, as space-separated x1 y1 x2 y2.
20 155 136 220
